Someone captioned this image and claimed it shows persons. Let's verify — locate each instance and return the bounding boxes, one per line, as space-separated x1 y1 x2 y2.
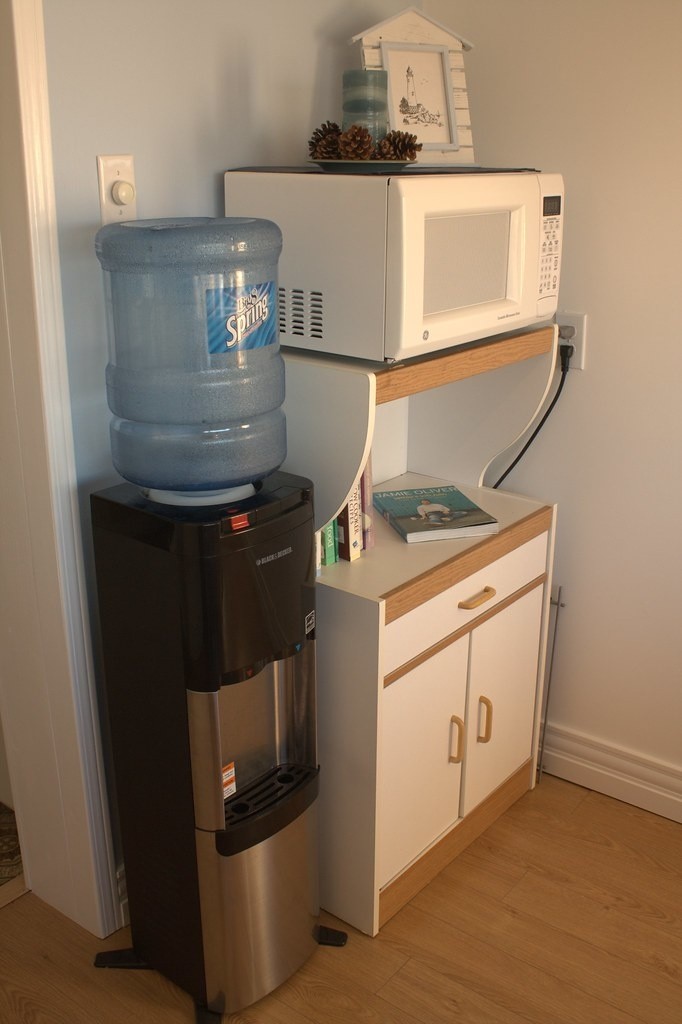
417 499 468 527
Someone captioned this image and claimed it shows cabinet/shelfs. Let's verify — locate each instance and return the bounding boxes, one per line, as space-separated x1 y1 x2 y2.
277 320 557 939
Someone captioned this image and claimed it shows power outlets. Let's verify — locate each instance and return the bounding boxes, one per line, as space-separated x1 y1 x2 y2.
555 313 587 369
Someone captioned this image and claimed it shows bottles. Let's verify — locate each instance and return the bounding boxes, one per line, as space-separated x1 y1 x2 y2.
96 212 288 495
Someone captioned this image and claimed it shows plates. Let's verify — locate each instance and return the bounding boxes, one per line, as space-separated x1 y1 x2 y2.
305 154 416 171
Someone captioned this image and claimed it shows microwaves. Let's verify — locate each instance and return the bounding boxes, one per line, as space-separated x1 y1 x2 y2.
223 169 564 364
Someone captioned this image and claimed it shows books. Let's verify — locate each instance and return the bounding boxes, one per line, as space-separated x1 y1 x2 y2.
320 450 376 567
372 486 500 543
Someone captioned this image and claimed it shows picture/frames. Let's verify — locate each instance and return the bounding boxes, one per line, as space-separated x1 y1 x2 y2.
347 4 476 167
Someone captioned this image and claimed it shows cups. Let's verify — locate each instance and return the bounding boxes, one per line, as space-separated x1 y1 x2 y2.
342 68 388 140
